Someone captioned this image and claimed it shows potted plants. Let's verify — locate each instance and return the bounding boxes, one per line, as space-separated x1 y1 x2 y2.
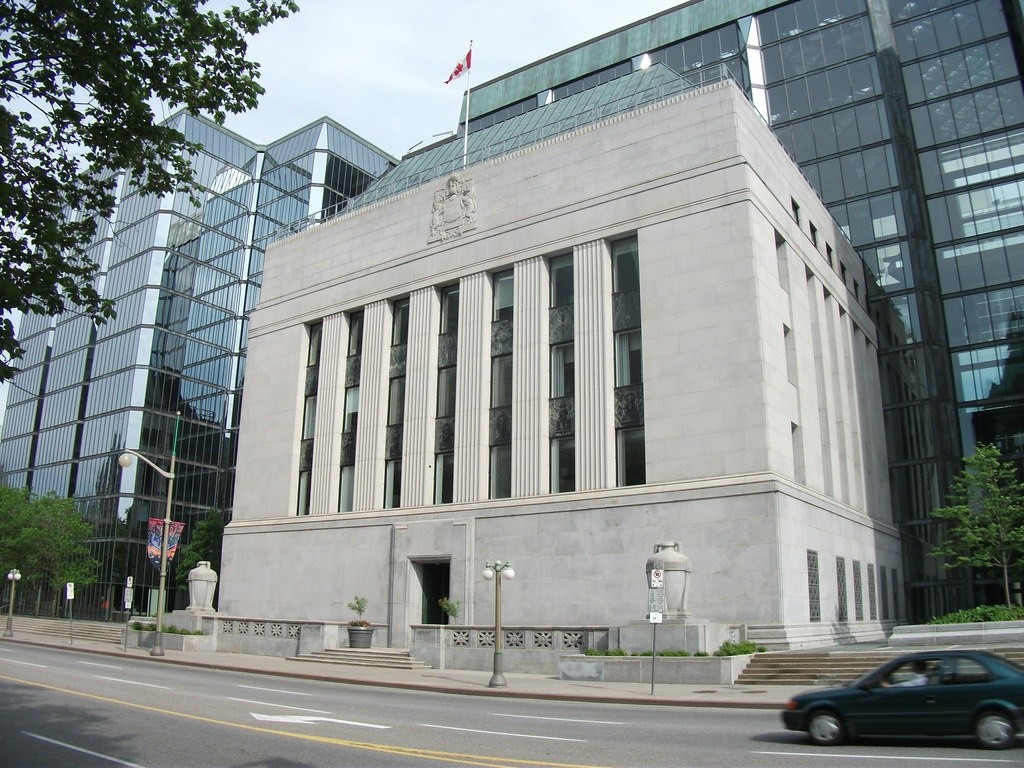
346 595 374 648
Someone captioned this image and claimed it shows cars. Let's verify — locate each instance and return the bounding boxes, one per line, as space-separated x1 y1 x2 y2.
782 651 1024 749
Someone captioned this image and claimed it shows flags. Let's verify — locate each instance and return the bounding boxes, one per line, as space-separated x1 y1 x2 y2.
446 49 471 84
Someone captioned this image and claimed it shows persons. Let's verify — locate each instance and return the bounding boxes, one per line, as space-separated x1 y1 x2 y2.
882 662 929 690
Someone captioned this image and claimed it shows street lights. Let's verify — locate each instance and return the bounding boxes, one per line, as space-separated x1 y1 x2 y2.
481 560 515 686
2 569 21 637
118 450 174 656
